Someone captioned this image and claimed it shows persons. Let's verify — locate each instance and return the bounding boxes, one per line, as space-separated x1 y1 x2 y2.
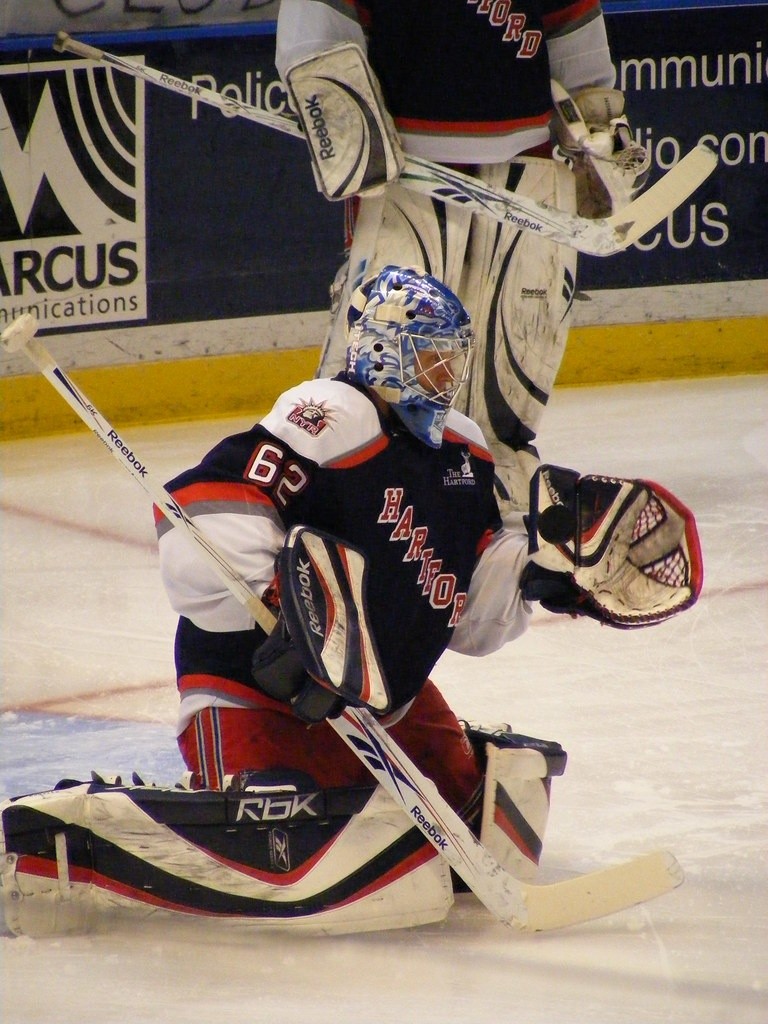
276 1 650 536
1 264 704 933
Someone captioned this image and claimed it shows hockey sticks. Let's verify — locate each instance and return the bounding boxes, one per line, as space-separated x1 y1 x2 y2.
0 310 687 931
54 28 720 258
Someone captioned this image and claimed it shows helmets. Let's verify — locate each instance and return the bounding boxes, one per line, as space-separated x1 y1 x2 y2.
346 266 473 448
